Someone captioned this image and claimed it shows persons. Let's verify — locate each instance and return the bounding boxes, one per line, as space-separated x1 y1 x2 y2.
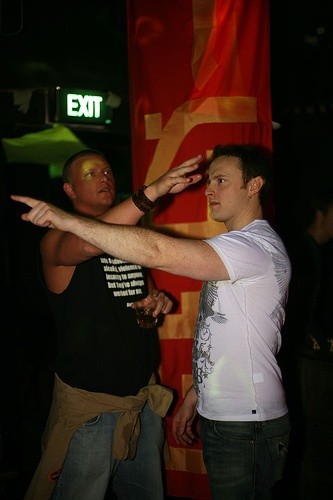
24 150 204 500
11 144 292 500
290 195 333 500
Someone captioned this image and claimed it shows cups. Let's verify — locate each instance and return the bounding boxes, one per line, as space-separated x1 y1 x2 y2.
135 298 158 328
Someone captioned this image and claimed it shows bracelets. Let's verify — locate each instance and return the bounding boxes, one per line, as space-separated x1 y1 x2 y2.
132 185 159 212
